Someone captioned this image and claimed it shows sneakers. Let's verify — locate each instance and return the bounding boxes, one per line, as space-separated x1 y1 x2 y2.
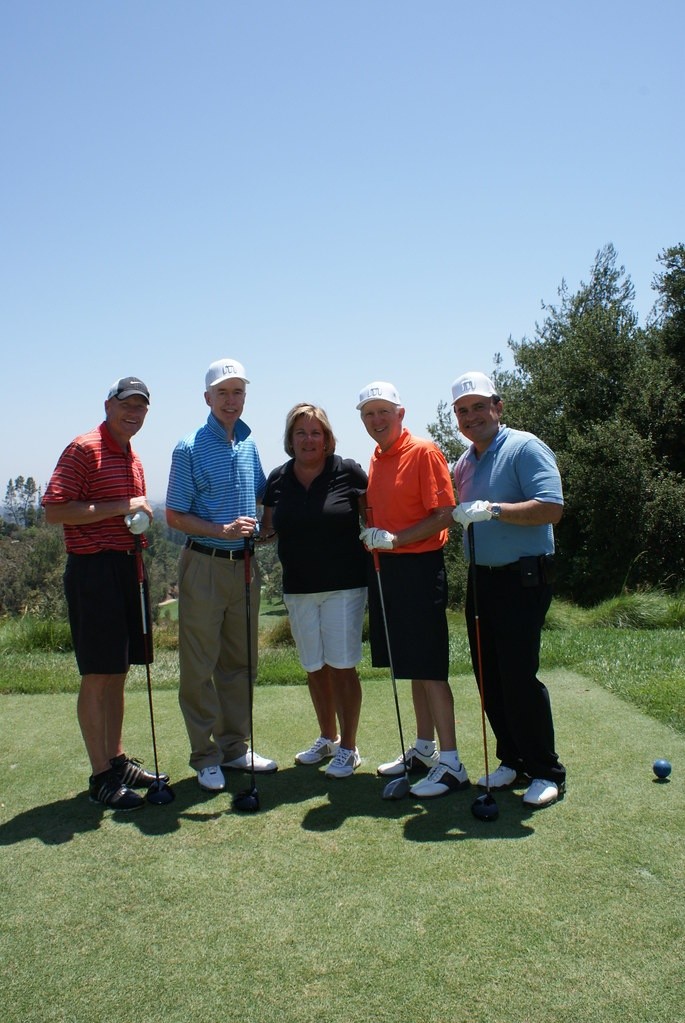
110 757 170 788
88 773 145 812
294 735 341 764
377 745 440 776
325 746 361 779
198 765 225 789
220 748 278 772
523 779 566 807
410 763 471 797
477 765 532 790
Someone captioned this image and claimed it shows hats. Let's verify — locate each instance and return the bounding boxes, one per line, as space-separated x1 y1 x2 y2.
356 382 401 410
451 372 497 405
107 376 151 405
205 359 250 390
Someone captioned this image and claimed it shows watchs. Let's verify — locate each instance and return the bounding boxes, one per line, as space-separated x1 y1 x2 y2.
490 502 501 521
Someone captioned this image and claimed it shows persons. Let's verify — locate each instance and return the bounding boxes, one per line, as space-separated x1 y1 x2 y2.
440 372 565 808
260 403 370 780
165 360 277 789
357 382 471 799
39 375 171 810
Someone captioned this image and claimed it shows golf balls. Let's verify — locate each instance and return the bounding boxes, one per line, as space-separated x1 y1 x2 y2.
653 759 672 778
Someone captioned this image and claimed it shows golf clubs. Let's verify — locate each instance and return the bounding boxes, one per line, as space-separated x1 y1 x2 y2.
232 533 262 813
134 531 178 805
364 506 411 801
465 519 500 822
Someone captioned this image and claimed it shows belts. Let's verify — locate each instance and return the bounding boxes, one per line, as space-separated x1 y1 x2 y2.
184 537 256 562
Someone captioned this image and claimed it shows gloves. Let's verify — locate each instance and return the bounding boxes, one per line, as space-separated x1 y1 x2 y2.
451 500 493 530
124 511 150 534
359 527 394 549
